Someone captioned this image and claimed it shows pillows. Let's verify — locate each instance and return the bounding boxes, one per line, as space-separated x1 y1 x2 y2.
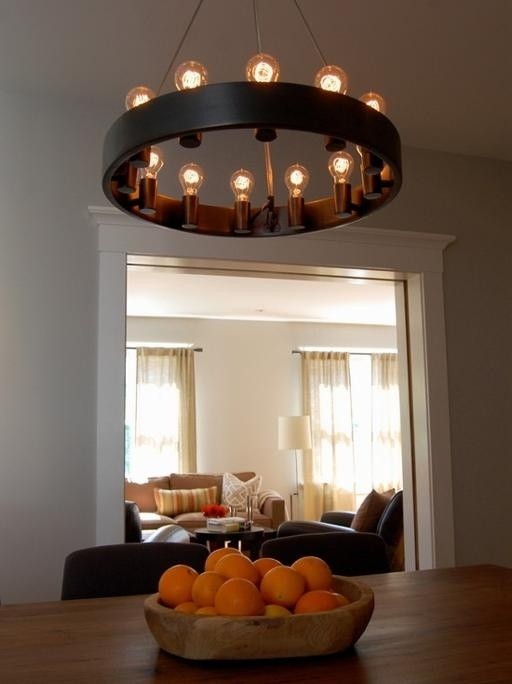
221 472 262 512
152 485 220 517
350 488 395 532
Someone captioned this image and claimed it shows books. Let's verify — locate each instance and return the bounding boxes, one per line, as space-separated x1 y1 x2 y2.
207 516 246 532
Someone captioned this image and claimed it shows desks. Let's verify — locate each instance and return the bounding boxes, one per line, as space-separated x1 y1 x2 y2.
1 562 512 684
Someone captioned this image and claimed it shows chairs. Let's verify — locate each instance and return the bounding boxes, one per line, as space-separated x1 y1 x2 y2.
125 499 191 544
278 488 403 566
258 532 390 577
60 542 210 601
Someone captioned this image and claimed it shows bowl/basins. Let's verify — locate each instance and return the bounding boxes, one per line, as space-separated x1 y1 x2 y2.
143 574 374 661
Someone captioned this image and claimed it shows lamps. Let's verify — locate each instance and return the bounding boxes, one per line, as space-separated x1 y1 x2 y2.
277 414 313 523
101 0 404 239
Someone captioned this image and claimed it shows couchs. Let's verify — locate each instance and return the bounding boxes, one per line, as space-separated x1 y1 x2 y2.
125 472 285 529
